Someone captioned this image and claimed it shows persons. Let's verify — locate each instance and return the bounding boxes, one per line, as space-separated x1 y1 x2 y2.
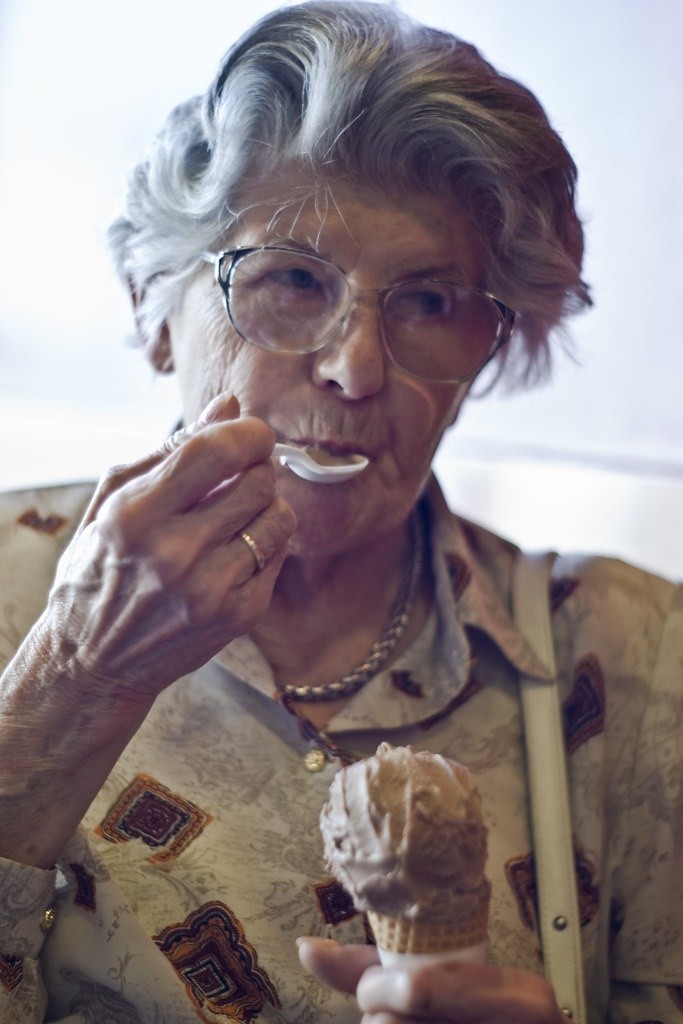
0 0 683 1024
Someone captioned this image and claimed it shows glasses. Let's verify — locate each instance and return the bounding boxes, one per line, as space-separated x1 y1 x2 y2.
200 244 521 384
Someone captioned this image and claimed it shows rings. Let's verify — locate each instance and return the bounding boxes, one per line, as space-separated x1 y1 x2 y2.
241 531 267 571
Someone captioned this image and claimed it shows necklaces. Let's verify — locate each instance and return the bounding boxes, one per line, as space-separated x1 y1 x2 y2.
283 514 421 699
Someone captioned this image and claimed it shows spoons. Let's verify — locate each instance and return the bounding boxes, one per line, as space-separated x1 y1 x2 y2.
170 429 370 485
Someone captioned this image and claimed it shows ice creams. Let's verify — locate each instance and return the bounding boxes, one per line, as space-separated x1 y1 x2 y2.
318 741 490 966
